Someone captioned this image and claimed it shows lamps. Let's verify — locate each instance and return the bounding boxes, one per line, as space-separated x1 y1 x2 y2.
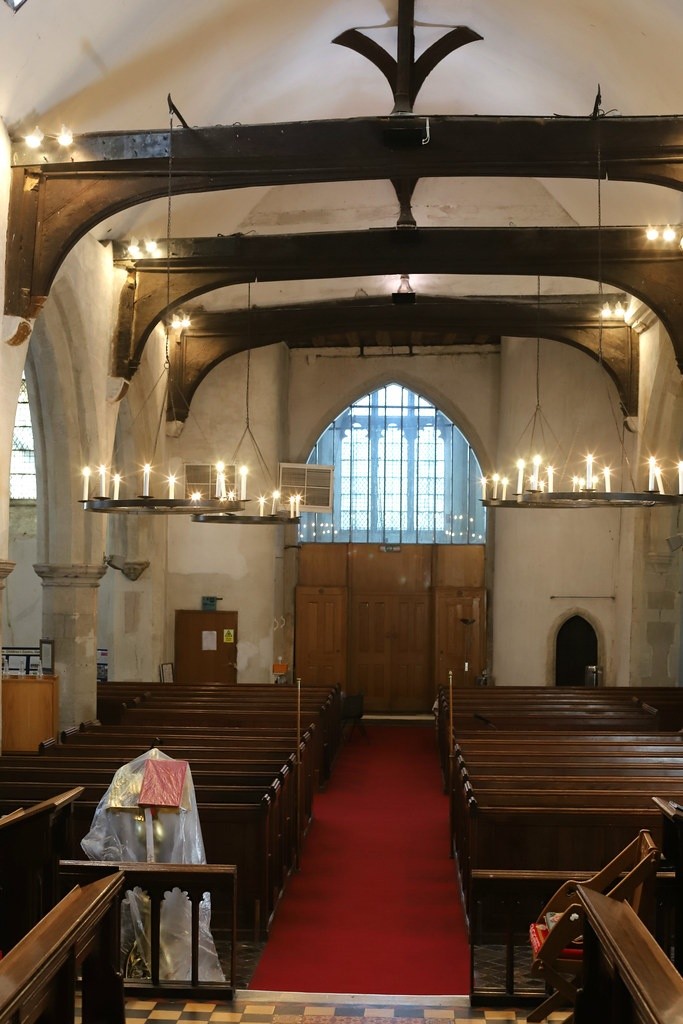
79 83 683 524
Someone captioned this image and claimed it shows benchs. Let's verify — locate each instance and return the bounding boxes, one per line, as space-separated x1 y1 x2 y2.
0 681 342 941
437 684 683 946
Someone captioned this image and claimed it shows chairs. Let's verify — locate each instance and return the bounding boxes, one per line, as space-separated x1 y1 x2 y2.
527 829 660 1024
342 694 370 745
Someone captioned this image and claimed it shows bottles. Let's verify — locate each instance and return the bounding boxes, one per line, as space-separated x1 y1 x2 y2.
3 658 43 679
